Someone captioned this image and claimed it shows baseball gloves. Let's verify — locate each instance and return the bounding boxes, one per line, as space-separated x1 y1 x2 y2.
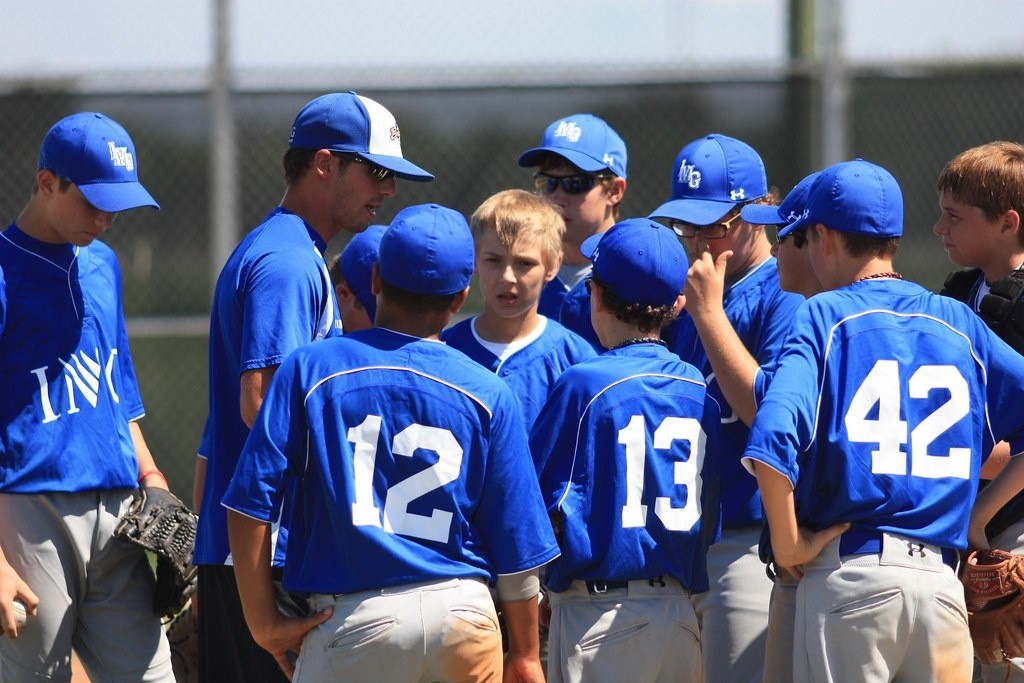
112 484 200 628
960 547 1023 683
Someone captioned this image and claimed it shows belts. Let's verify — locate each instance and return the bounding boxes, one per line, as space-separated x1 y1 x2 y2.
839 533 954 565
587 576 666 593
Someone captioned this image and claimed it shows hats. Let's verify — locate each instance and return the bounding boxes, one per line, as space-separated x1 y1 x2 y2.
289 90 436 181
741 171 821 224
779 157 903 238
378 202 473 295
580 217 689 313
519 113 626 181
341 223 393 323
647 133 766 225
41 109 160 212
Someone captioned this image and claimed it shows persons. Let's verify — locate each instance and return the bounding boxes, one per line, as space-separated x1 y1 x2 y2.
740 169 820 683
438 188 599 683
0 110 197 683
517 112 630 357
328 223 390 336
529 216 723 683
191 91 436 683
741 156 1024 683
650 132 807 683
931 140 1024 683
219 204 562 683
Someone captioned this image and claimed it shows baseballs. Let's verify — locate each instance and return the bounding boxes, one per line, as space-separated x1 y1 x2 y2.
2 597 29 630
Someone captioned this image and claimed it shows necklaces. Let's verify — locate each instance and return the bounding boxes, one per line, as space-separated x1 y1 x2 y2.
610 337 668 350
849 273 902 284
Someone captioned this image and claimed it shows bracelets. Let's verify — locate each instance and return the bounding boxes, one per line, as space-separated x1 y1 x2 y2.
138 470 169 486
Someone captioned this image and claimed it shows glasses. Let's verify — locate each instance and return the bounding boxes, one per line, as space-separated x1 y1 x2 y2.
669 211 742 239
532 171 617 195
312 150 396 181
